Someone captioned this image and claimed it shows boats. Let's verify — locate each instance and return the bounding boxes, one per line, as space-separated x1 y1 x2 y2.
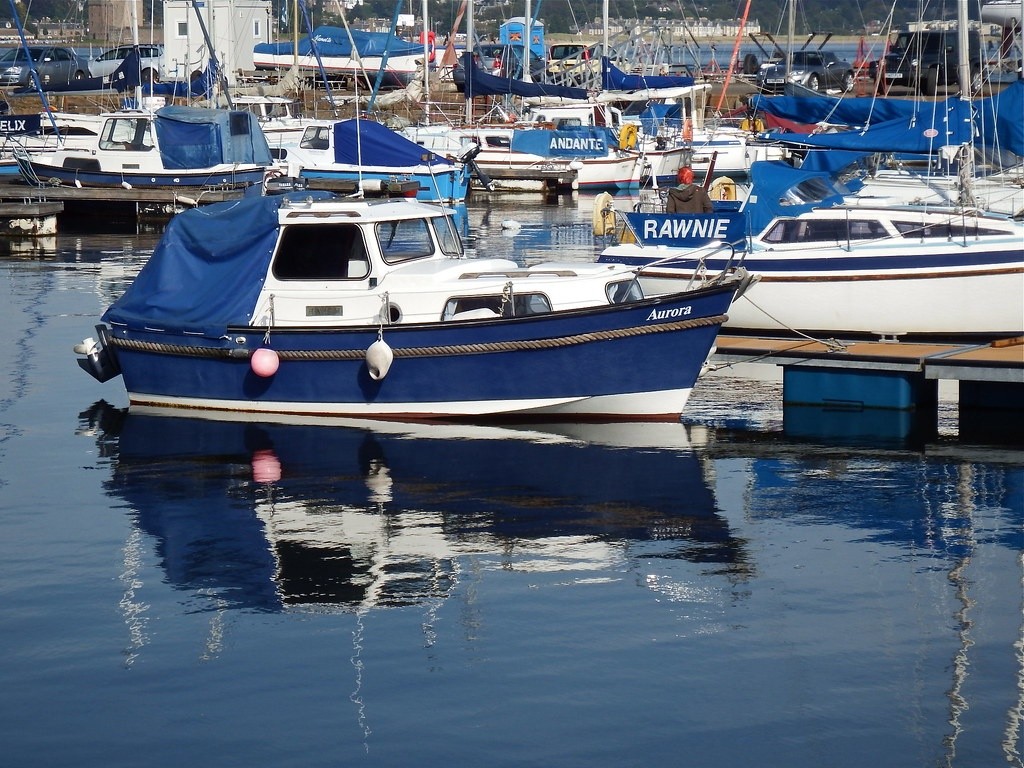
0 0 1024 343
71 46 763 426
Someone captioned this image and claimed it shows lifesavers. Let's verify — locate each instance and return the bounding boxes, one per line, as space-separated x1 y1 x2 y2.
594 192 615 236
743 118 764 132
43 105 58 112
683 119 693 142
428 37 435 60
711 176 737 201
620 124 636 149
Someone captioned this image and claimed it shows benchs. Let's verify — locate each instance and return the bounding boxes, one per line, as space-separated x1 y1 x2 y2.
236 77 266 88
315 80 345 91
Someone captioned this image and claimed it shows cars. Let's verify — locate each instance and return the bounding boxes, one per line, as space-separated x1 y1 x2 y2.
546 40 632 89
0 44 89 94
451 43 549 93
756 49 856 95
87 43 163 87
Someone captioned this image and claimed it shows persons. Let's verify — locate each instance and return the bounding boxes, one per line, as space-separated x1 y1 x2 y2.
666 167 712 213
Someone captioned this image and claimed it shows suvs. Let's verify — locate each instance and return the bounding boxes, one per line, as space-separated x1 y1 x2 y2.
868 28 986 97
